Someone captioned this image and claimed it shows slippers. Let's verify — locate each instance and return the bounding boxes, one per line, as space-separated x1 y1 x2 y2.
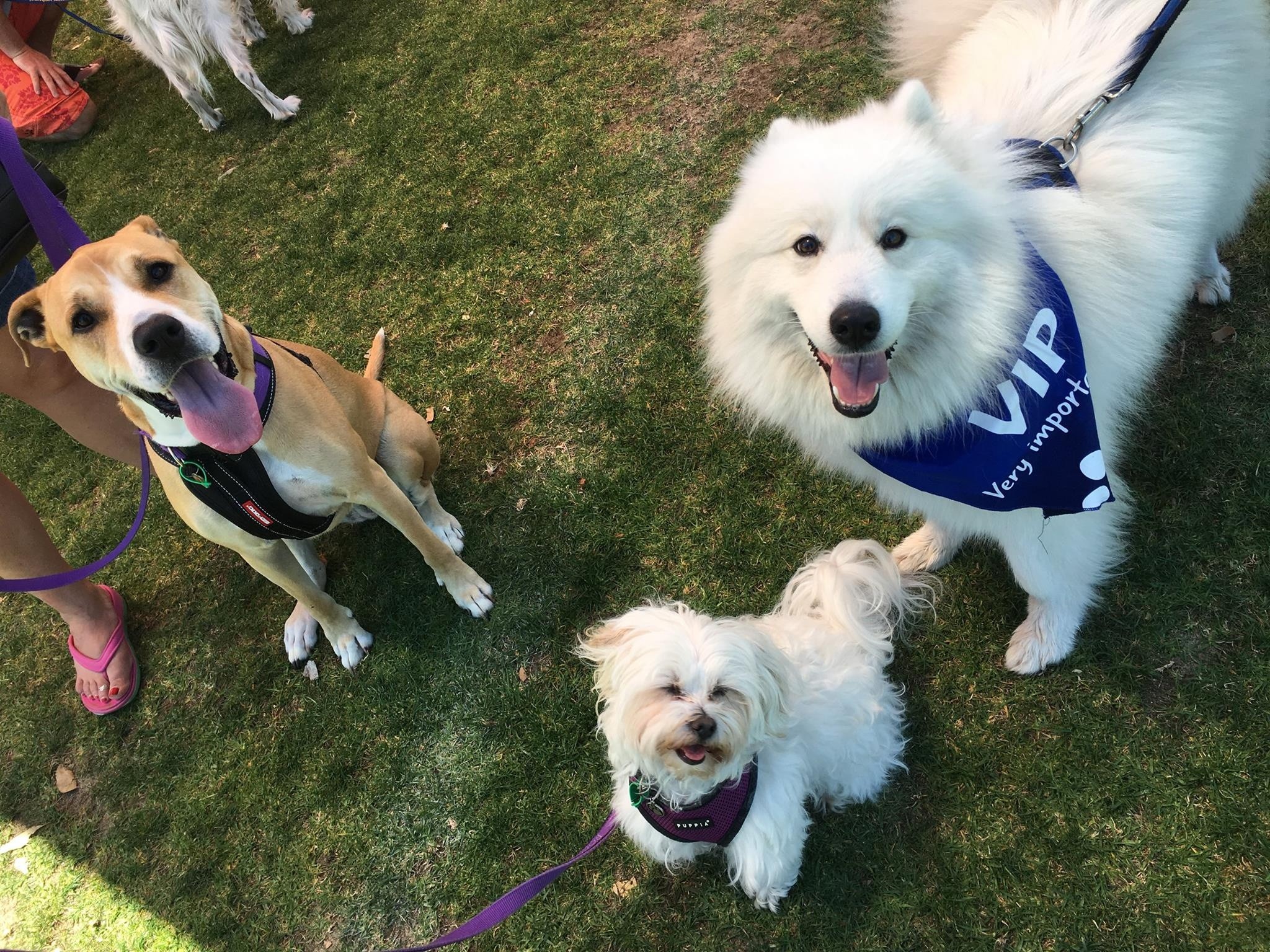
68 584 138 716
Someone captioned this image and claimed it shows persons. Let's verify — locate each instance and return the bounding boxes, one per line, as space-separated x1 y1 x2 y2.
0 0 103 142
0 146 156 715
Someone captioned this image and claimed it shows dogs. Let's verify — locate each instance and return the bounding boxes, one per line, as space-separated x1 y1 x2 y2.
4 211 497 676
100 1 320 136
685 0 1269 680
561 533 957 917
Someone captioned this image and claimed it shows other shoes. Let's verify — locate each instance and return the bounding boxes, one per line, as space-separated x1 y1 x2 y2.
64 57 106 82
